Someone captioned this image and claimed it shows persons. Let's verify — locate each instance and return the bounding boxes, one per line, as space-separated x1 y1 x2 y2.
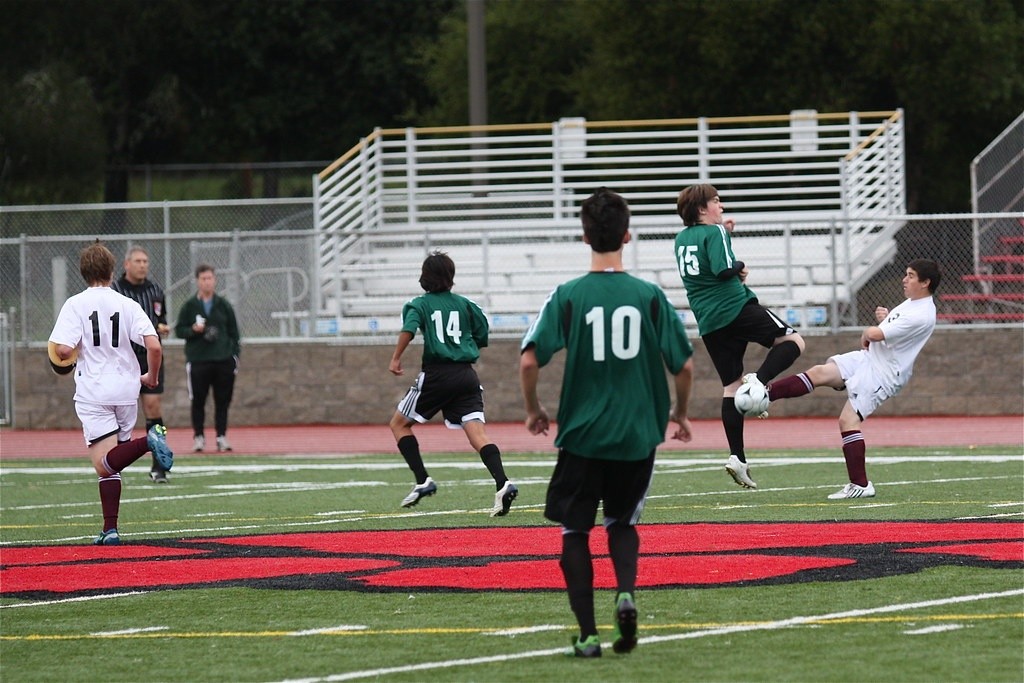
108 244 170 483
174 264 241 453
46 242 176 546
675 184 806 487
388 253 518 517
518 188 694 657
762 258 942 498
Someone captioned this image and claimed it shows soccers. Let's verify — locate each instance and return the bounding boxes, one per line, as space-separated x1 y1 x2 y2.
733 381 771 416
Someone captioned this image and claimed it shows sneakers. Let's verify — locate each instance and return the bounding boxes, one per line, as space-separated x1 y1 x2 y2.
562 634 602 659
146 423 173 470
490 481 519 517
724 456 757 488
149 472 168 484
191 435 205 451
216 435 232 452
827 480 876 499
94 528 119 544
741 372 770 419
611 592 638 654
400 477 437 508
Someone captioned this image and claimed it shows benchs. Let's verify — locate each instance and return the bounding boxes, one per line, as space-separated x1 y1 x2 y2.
270 245 890 326
936 217 1024 324
